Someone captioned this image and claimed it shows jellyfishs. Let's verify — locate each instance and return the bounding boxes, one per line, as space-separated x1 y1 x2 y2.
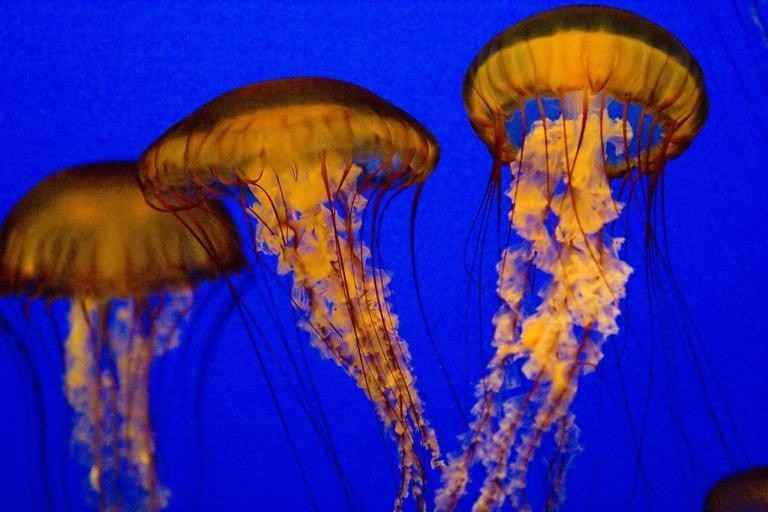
431 5 764 512
135 75 478 512
0 160 250 511
